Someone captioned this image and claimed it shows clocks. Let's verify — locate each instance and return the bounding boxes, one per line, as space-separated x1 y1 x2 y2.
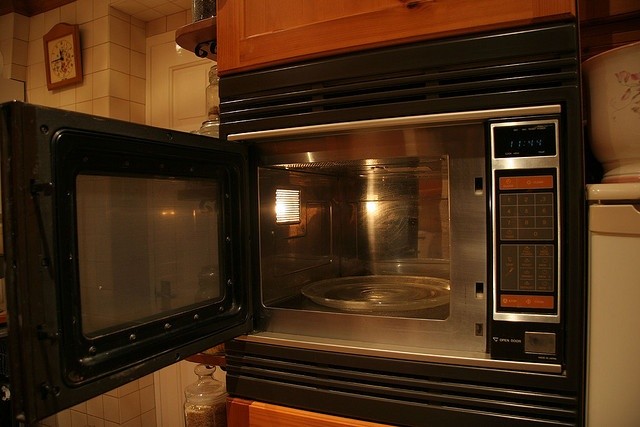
42 22 83 90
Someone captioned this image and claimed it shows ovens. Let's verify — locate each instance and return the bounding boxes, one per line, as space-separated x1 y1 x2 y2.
0 13 588 427
